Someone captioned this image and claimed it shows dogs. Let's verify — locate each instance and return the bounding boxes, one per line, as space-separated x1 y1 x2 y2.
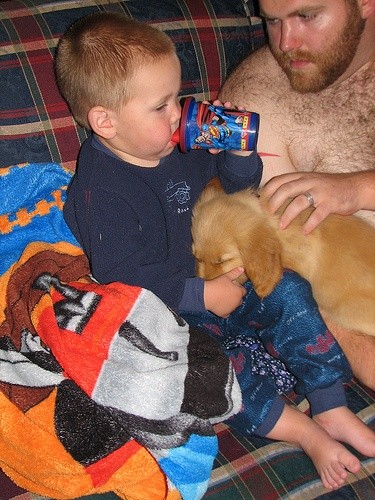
189 174 375 393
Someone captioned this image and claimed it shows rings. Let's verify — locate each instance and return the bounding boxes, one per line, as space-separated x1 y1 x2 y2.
304 191 314 207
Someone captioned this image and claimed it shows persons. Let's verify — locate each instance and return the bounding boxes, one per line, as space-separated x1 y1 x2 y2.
216 0 375 235
53 10 375 493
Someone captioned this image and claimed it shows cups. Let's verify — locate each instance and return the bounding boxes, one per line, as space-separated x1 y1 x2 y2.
173 96 260 154
